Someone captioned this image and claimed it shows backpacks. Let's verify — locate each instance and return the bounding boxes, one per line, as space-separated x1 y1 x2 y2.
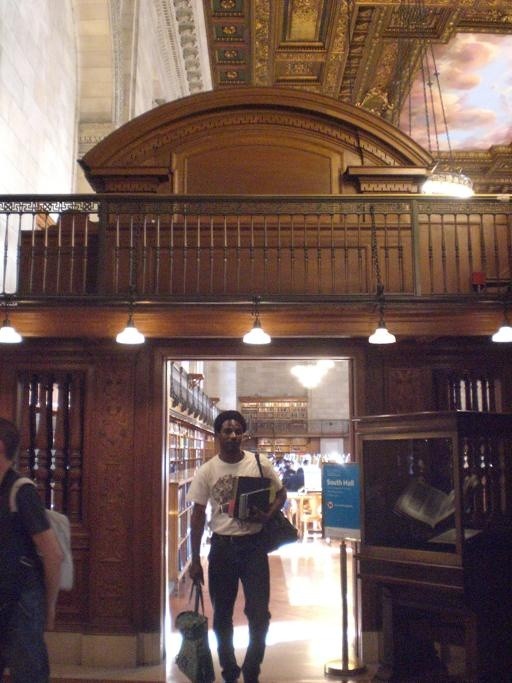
9 476 73 594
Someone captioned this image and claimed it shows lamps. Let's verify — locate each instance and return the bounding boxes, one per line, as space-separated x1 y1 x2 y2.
0 294 22 343
368 295 396 344
242 296 271 345
419 0 474 199
491 295 512 342
116 296 144 345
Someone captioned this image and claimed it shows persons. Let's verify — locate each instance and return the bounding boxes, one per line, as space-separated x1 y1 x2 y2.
187 410 287 683
0 418 64 682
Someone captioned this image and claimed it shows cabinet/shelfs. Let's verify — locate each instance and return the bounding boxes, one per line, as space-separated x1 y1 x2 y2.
258 437 310 460
169 417 214 473
243 403 307 433
351 411 512 683
168 468 197 592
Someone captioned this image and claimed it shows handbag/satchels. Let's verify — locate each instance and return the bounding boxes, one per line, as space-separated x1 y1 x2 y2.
174 612 215 682
262 508 298 553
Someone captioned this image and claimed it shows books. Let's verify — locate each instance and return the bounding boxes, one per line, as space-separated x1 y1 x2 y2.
242 401 305 432
229 475 271 518
170 421 206 472
239 487 275 520
